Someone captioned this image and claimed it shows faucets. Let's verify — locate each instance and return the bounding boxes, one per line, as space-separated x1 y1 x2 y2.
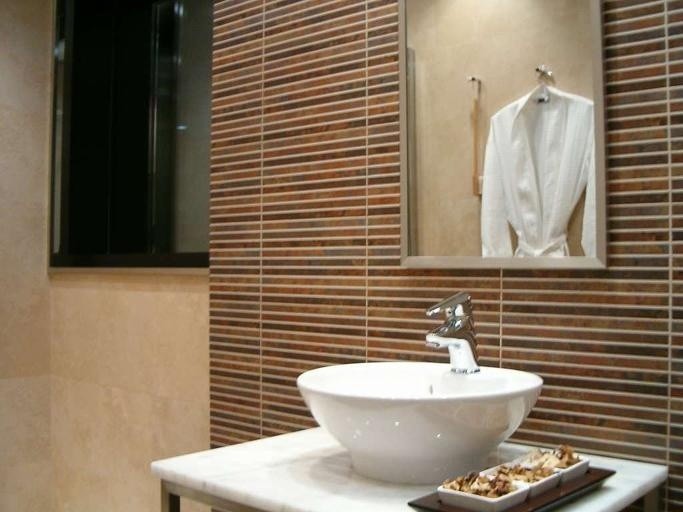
425 291 479 374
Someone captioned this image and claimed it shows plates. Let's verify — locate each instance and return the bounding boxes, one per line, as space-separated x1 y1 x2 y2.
482 460 559 499
511 447 590 485
406 448 617 512
435 471 529 510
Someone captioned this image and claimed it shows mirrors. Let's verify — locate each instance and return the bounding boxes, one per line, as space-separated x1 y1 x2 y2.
398 0 608 271
51 1 211 274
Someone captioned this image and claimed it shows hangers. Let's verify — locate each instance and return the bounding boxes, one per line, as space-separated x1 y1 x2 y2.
528 69 557 103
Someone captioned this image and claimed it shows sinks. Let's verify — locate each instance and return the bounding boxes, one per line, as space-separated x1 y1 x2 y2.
296 361 544 484
